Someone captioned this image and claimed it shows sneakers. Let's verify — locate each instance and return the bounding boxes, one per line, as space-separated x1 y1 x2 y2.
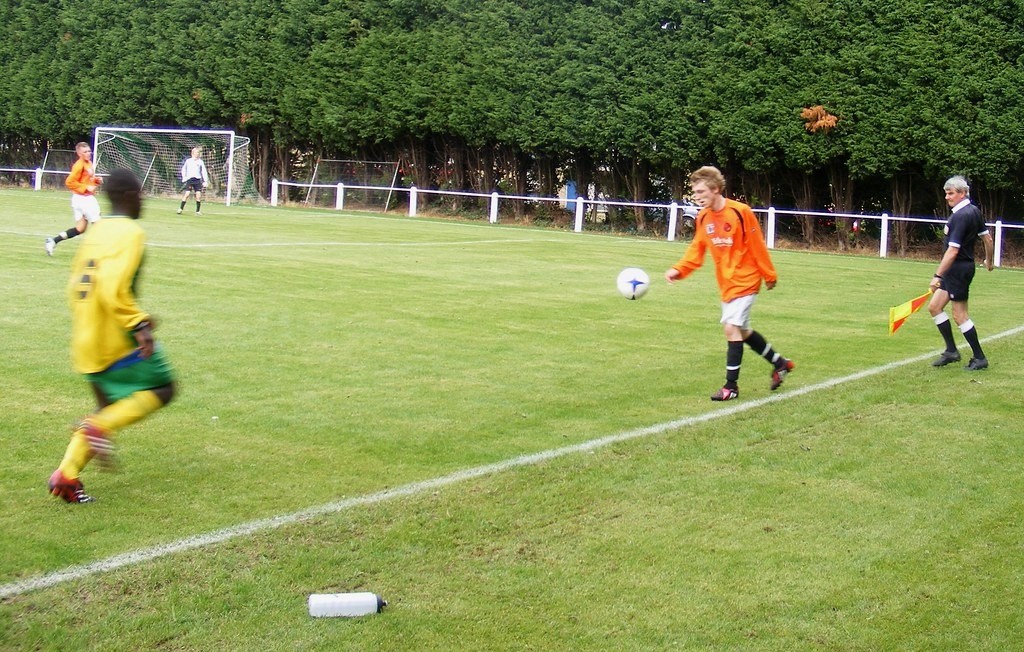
84 421 112 461
48 471 94 503
771 359 795 389
46 238 57 256
963 356 988 370
932 349 960 366
711 386 738 400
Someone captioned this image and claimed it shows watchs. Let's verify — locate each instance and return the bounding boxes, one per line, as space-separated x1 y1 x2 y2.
934 274 941 278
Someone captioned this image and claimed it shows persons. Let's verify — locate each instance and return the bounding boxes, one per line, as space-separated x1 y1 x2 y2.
930 177 993 371
666 166 794 401
45 142 103 257
177 148 208 217
48 168 175 503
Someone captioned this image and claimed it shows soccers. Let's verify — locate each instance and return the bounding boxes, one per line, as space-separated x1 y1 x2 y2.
617 267 651 299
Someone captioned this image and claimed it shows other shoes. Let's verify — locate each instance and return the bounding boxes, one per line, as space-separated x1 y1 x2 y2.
196 212 202 216
177 209 182 214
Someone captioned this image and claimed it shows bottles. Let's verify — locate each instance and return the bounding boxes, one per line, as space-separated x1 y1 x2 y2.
308 592 386 617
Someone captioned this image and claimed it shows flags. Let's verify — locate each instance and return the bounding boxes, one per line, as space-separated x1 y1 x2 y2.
890 288 932 336
